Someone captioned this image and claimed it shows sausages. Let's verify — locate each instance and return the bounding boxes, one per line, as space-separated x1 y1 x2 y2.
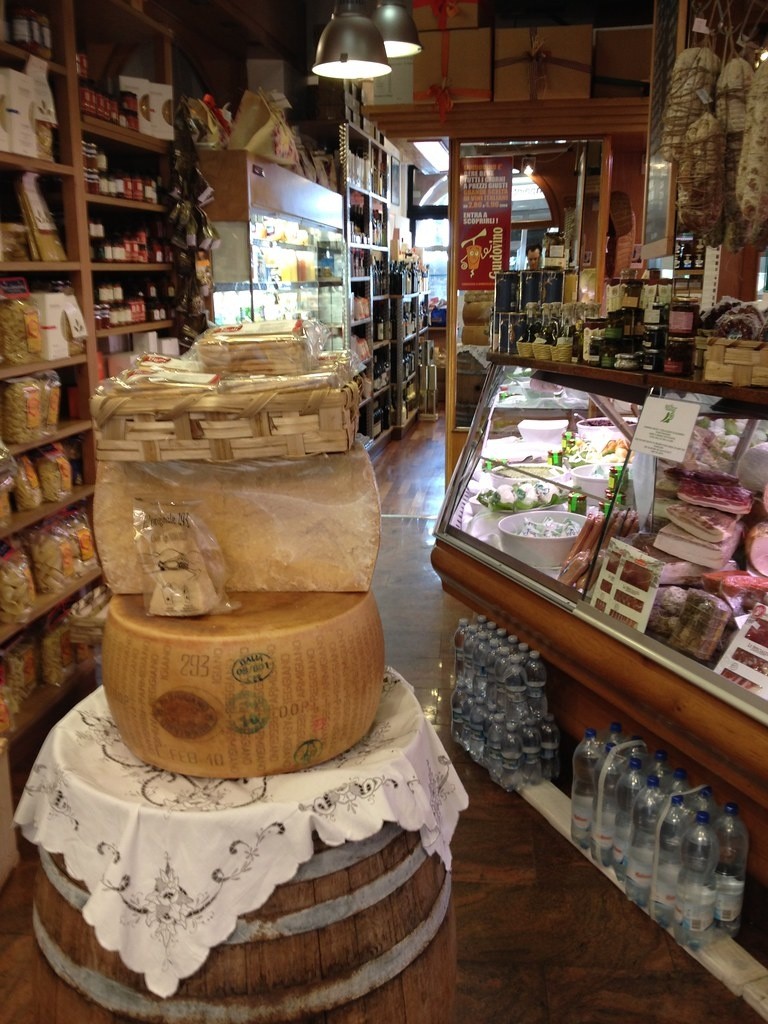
556 508 640 589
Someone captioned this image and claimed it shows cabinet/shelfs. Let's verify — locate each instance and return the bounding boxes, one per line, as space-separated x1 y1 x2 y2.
293 120 431 460
429 328 445 411
0 0 178 771
604 231 745 353
197 149 350 350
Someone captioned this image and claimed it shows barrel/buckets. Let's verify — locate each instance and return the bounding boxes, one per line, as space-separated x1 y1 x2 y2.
26 822 460 1024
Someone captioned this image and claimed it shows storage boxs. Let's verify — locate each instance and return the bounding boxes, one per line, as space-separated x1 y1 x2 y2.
97 332 181 380
318 74 400 158
118 76 174 141
373 0 596 105
0 67 38 159
29 291 69 360
246 58 306 122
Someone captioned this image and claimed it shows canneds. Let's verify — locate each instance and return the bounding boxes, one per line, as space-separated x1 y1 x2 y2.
76 45 177 332
0 0 52 61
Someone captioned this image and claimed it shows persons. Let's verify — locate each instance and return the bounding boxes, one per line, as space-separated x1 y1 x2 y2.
527 244 540 270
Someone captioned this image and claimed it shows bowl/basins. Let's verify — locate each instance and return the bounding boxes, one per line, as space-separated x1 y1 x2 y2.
576 417 638 451
491 462 569 490
498 511 588 569
571 463 631 507
517 420 569 445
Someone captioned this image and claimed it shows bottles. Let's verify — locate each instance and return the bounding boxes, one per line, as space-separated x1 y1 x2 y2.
450 615 561 793
351 204 429 439
570 721 750 952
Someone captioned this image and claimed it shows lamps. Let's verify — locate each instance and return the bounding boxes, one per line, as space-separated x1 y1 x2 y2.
311 0 393 79
372 0 421 58
406 139 449 174
